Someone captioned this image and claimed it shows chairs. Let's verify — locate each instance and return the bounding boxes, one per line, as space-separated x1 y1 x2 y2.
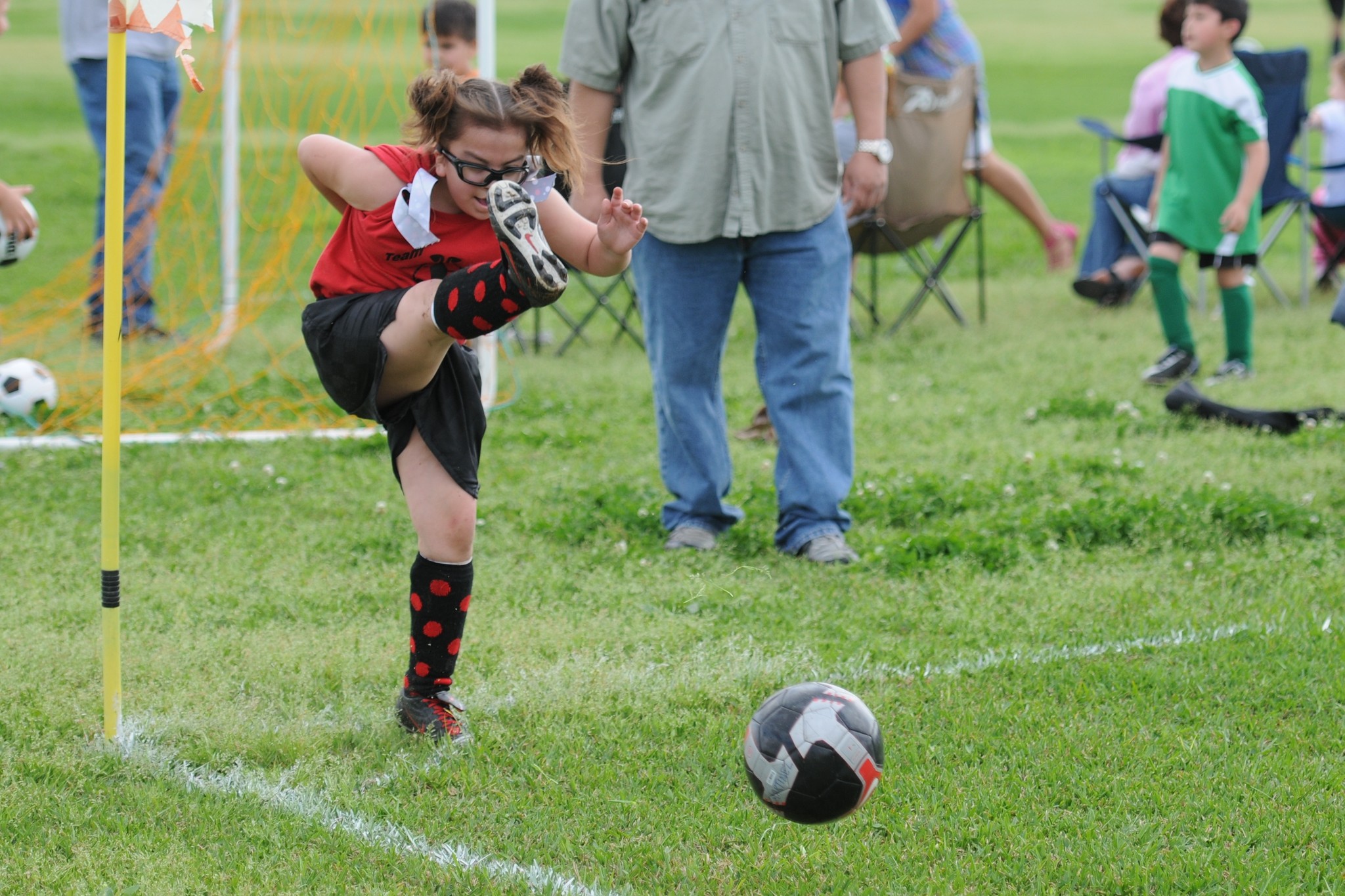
515 66 987 358
1081 46 1309 309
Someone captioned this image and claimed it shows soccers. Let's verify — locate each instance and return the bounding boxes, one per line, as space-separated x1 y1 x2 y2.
1 357 58 428
743 681 884 826
0 197 41 267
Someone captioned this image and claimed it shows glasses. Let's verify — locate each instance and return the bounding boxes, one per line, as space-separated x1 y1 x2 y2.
435 145 530 188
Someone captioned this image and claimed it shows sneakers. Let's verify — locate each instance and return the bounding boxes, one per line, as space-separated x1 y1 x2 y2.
1205 357 1246 386
1139 349 1198 387
484 180 570 308
665 522 717 550
394 688 473 749
799 535 857 565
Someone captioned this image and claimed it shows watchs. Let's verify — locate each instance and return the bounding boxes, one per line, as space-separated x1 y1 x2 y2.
856 139 893 163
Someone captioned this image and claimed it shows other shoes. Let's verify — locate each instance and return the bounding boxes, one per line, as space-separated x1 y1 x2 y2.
1043 220 1078 273
1073 265 1130 303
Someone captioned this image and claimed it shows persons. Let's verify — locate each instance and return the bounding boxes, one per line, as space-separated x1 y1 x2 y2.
832 62 894 229
1074 0 1198 306
541 76 628 203
420 0 478 84
1143 0 1269 384
562 0 901 563
60 0 180 341
885 0 1078 272
1306 55 1345 288
298 64 647 746
0 0 39 240
